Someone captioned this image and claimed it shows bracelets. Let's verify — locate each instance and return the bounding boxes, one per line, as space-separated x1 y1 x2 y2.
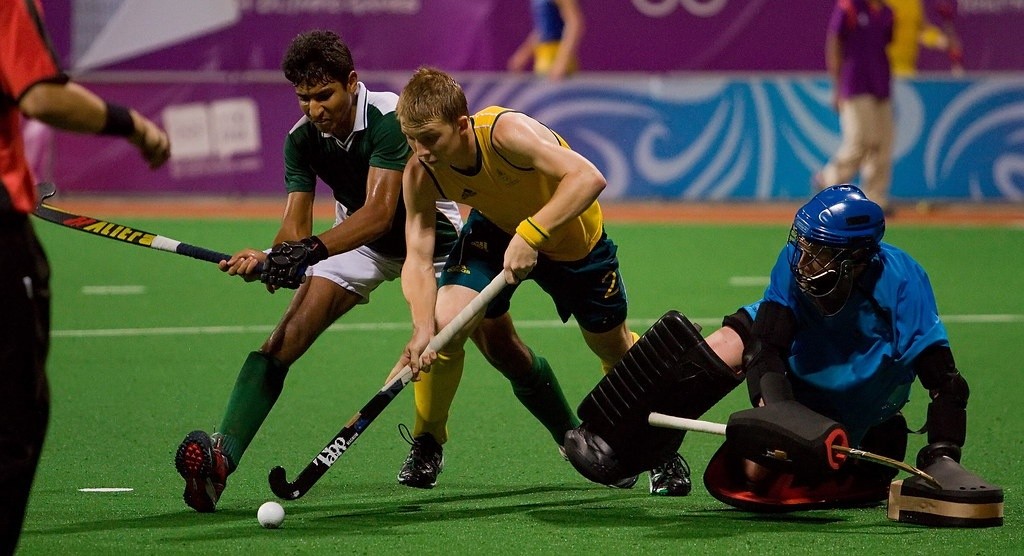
301 235 329 266
516 216 551 251
97 101 135 138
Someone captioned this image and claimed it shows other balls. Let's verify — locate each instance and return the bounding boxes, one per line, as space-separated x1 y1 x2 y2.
257 502 285 529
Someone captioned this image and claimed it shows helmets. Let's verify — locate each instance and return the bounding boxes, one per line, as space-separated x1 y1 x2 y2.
786 183 885 320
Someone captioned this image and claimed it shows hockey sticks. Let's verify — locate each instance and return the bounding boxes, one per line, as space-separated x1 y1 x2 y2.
647 411 944 490
267 269 508 501
30 180 307 284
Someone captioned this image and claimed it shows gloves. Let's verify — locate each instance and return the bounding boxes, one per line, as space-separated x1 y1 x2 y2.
259 236 328 294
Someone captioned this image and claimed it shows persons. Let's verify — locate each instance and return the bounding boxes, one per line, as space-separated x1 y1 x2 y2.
508 0 587 83
0 0 170 556
384 66 692 497
566 183 1005 529
813 0 950 217
175 30 639 512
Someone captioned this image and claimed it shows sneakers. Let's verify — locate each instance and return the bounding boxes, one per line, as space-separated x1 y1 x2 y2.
607 474 639 489
398 423 444 489
647 452 691 496
175 425 234 512
